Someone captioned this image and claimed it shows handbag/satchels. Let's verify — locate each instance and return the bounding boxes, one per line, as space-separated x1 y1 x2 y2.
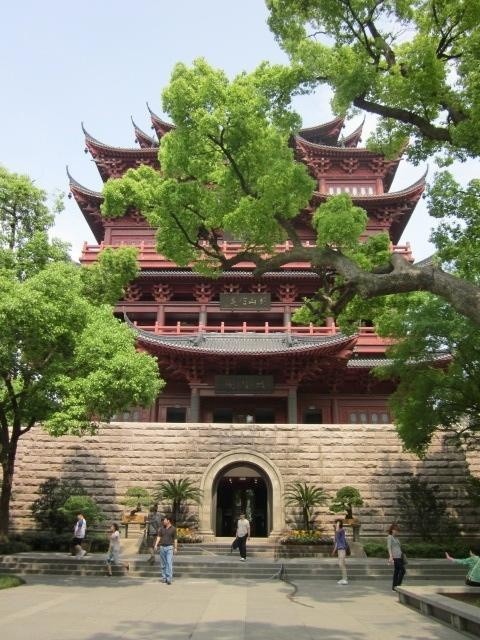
402 552 408 565
346 545 351 556
232 538 241 550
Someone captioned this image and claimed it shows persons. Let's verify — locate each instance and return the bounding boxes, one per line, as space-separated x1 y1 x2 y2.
236 512 251 562
102 522 130 578
330 518 349 586
386 525 406 592
66 511 88 557
154 515 178 586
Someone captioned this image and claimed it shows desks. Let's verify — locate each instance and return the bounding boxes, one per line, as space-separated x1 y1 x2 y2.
120 519 158 547
336 523 359 541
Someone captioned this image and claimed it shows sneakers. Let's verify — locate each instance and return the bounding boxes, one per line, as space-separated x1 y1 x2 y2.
337 579 349 585
125 562 130 573
75 549 87 557
162 578 172 584
240 558 247 562
104 573 111 578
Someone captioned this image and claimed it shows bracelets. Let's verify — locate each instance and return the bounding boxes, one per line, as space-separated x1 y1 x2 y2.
174 546 178 550
